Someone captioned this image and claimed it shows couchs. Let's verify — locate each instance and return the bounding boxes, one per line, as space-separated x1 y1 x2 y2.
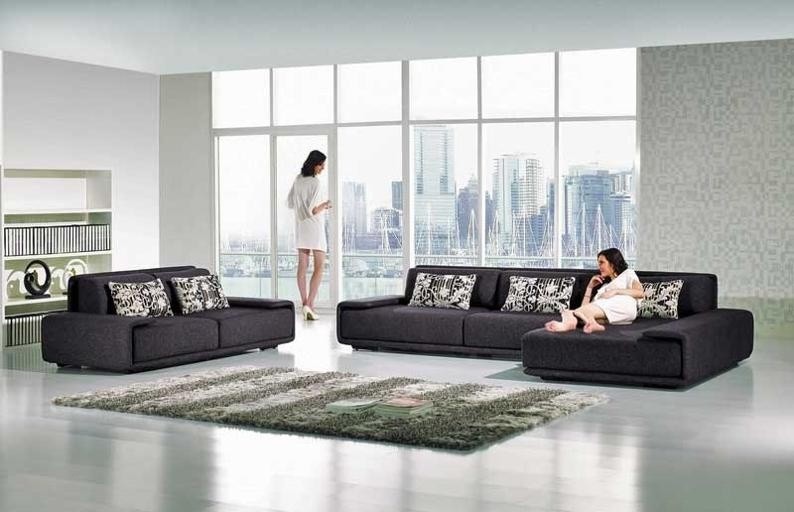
336 264 755 389
40 265 295 374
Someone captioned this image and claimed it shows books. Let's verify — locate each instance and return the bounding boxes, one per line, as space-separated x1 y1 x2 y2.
5 310 45 347
2 222 110 257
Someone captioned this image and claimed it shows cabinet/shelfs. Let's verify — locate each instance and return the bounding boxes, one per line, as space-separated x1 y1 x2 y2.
2 209 114 348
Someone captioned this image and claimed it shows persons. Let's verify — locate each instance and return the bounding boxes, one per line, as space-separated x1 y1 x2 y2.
543 246 646 333
284 148 335 322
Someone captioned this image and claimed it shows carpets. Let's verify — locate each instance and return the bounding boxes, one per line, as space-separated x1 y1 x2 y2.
52 365 613 451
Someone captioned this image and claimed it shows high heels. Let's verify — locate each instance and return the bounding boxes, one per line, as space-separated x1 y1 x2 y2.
303 305 319 320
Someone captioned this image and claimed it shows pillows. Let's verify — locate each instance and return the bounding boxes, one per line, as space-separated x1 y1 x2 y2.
637 275 688 321
499 275 575 315
108 277 175 319
408 272 480 312
170 272 230 315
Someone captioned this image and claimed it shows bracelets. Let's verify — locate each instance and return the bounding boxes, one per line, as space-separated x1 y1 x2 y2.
583 294 591 298
